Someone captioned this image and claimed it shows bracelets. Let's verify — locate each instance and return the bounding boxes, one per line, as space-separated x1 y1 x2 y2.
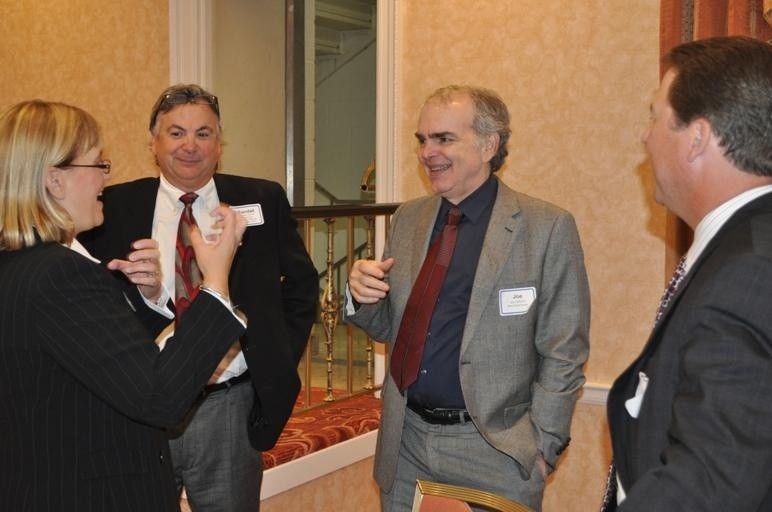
197 284 231 305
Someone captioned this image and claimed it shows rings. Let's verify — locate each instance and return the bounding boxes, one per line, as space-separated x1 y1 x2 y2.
235 239 243 247
154 263 161 276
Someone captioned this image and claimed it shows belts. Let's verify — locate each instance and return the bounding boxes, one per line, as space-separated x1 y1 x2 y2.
406 401 472 426
199 368 249 399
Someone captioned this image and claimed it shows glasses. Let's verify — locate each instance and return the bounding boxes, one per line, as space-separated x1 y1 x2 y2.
150 91 221 126
53 158 112 176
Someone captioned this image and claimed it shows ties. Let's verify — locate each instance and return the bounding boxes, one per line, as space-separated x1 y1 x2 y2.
388 204 464 397
173 191 204 333
598 251 686 512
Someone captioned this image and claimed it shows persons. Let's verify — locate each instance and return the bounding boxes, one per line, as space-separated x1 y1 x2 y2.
76 82 319 512
606 36 772 509
342 85 589 512
2 100 249 512
